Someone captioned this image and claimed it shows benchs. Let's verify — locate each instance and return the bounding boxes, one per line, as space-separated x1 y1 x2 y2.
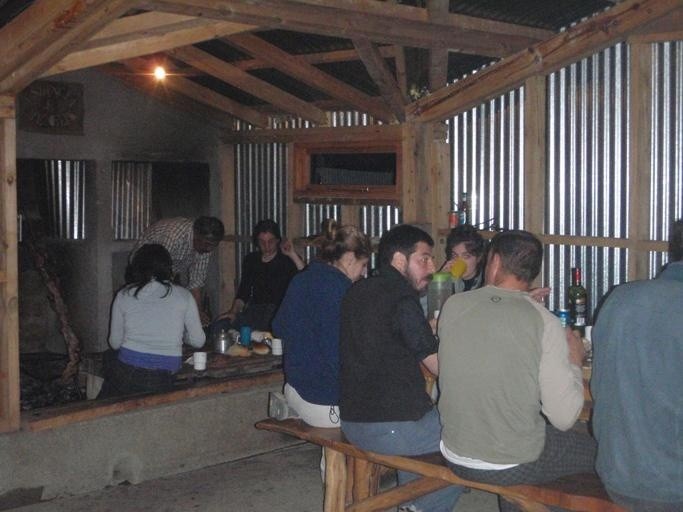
254 417 633 512
20 367 305 506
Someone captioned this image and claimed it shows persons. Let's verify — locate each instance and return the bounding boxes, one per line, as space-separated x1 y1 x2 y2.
108 243 206 392
438 225 487 293
339 224 442 455
128 217 225 327
589 219 683 511
270 218 373 428
215 219 307 332
437 231 596 486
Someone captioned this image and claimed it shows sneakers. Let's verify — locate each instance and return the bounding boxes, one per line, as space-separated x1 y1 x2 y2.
269 392 299 420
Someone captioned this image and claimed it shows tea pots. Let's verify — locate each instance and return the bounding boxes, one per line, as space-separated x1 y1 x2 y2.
207 329 242 354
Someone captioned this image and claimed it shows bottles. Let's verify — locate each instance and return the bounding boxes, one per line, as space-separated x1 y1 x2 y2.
554 308 571 330
448 211 458 229
568 267 586 337
458 192 469 225
427 273 452 323
240 326 251 346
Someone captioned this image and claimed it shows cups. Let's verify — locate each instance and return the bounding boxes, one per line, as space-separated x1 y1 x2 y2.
451 259 468 277
241 326 253 345
272 338 283 356
193 351 208 372
583 325 592 342
